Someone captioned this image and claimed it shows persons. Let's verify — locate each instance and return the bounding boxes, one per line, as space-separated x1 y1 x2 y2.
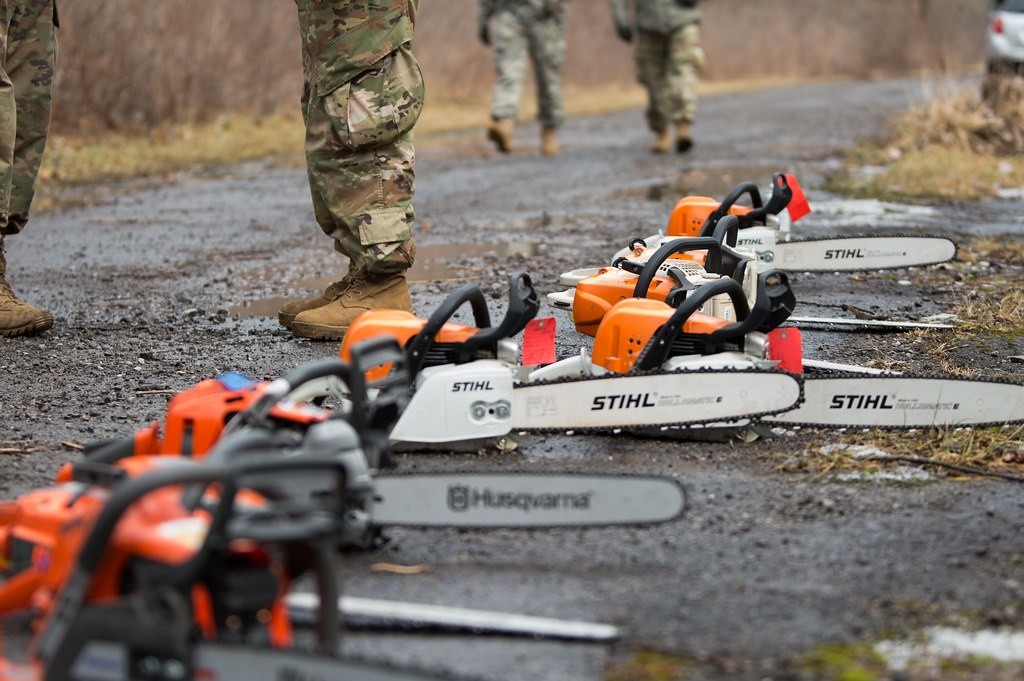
0 0 56 336
279 0 426 340
476 0 570 155
609 0 707 152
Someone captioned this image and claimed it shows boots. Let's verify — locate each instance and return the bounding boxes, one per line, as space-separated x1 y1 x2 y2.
0 241 53 336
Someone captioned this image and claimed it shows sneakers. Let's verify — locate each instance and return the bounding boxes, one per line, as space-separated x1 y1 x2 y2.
292 276 417 340
278 269 358 327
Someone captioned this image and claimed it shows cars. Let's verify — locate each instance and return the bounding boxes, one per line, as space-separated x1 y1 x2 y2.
987 0 1024 75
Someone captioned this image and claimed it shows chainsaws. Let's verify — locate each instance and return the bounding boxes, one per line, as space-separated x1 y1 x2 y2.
0 337 805 681
334 273 1024 444
545 171 957 338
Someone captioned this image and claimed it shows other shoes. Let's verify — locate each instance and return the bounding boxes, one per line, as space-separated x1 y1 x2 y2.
542 129 557 155
654 127 671 152
488 120 513 151
677 122 694 153
